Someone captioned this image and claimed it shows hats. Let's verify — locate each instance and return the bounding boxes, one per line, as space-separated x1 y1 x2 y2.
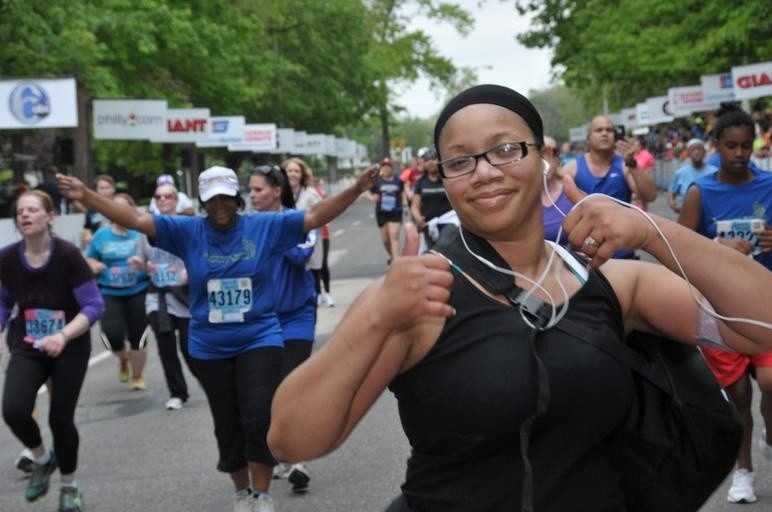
198 166 240 203
434 85 543 149
381 158 393 166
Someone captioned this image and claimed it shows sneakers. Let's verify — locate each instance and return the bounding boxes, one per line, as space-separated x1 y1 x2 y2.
317 292 335 306
166 397 183 410
235 462 310 512
727 467 757 503
15 449 83 512
120 360 145 389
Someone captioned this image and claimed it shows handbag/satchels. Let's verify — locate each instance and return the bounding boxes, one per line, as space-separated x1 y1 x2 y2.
625 331 742 512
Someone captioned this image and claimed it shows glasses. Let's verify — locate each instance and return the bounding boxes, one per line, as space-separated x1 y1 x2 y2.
156 195 171 198
438 141 538 178
256 165 277 182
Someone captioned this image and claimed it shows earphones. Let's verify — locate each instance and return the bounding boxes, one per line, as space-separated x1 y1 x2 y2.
542 157 551 175
